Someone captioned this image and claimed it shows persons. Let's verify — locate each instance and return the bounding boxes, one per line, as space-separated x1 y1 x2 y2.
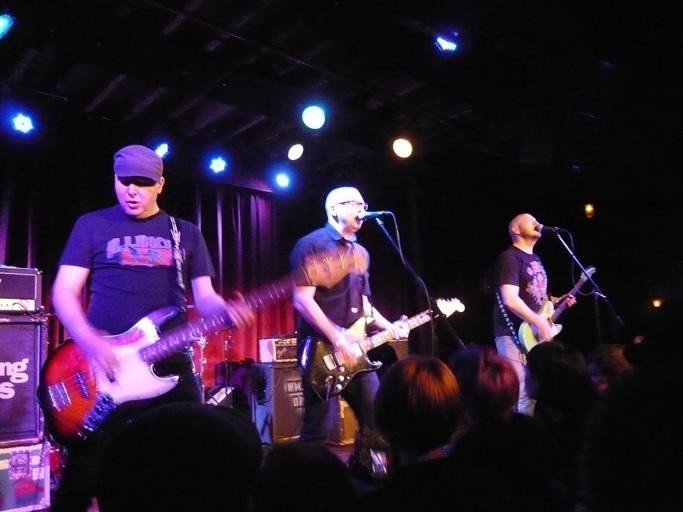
53 330 683 511
287 184 412 487
491 212 579 422
51 143 257 429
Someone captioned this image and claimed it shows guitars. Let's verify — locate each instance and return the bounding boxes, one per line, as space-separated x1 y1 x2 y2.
297 296 466 400
35 250 367 453
518 267 597 350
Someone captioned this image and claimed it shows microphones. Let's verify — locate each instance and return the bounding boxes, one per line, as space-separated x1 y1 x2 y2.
355 210 387 221
535 224 566 232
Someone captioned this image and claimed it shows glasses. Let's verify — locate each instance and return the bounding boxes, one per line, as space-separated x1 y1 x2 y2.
333 199 369 211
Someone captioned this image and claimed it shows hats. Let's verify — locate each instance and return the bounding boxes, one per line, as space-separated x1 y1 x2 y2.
112 145 163 183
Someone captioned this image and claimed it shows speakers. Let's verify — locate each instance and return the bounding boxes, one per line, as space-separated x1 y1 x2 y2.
0 314 49 449
252 361 311 443
